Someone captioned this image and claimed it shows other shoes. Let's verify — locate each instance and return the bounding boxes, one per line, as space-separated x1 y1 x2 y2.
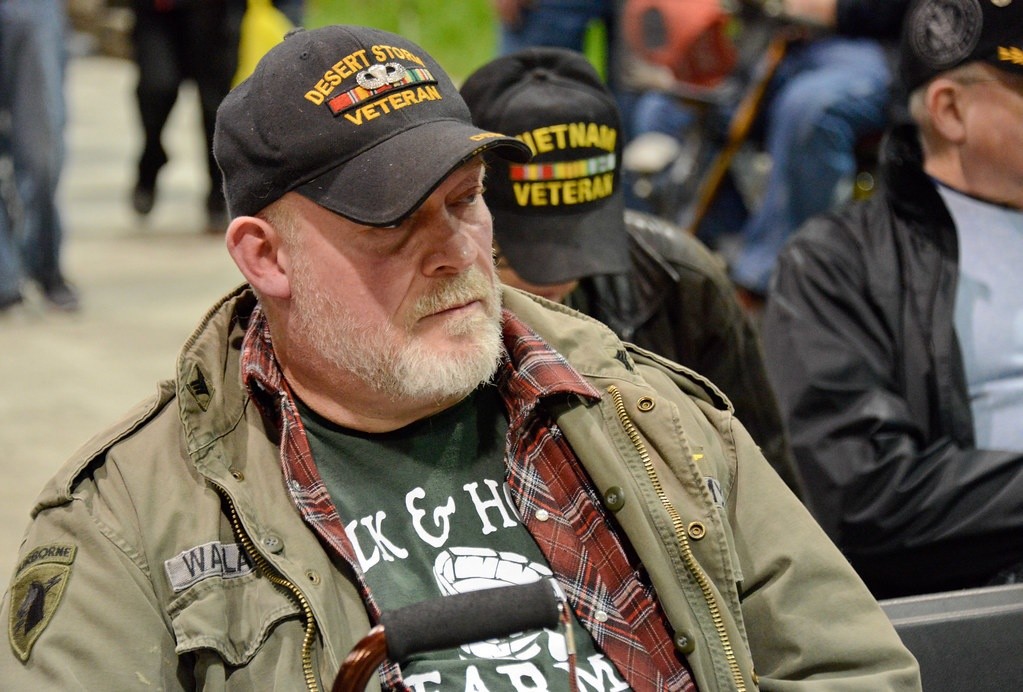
207 168 226 211
135 148 168 211
41 272 74 310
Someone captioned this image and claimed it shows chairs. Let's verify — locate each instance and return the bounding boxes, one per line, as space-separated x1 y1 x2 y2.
623 26 814 239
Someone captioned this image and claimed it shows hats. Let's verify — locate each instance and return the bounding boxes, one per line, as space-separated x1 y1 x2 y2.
211 24 534 227
460 46 635 286
901 0 1023 80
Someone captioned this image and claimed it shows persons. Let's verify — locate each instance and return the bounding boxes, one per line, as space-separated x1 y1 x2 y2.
761 0 1023 597
0 0 79 311
462 45 812 512
628 0 889 295
494 0 610 55
0 23 923 692
106 0 304 232
